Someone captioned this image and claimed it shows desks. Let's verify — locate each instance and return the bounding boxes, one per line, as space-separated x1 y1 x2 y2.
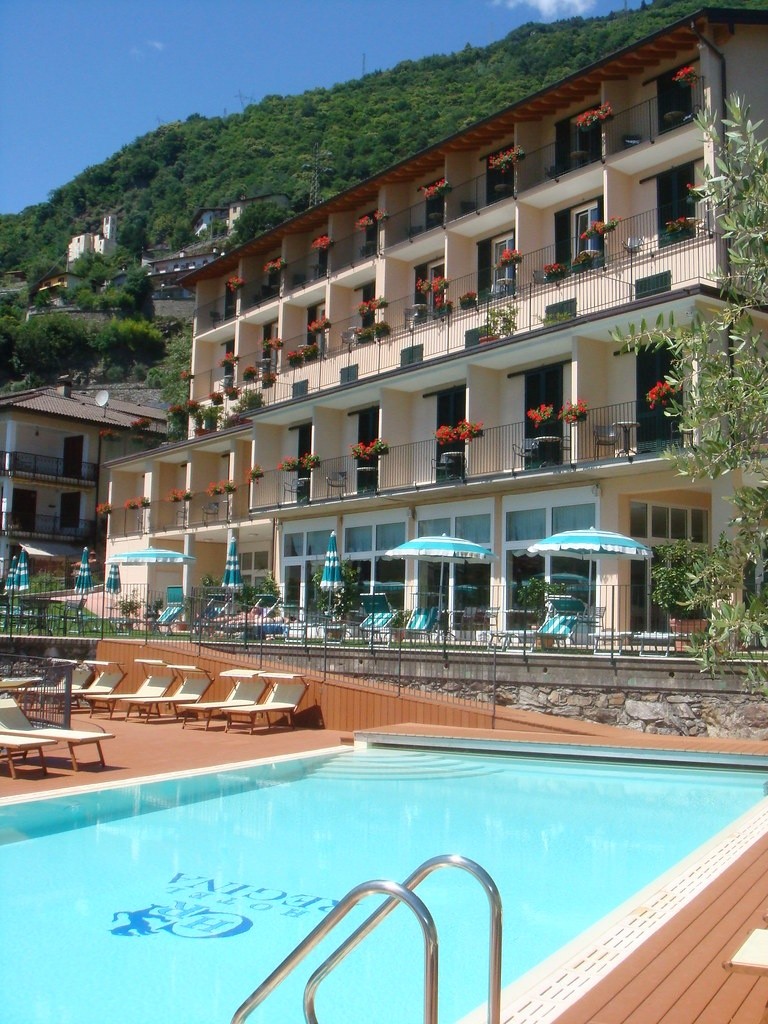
496 278 514 297
580 250 600 268
356 467 376 493
298 478 311 503
412 304 426 322
261 358 273 375
298 345 311 358
20 598 63 637
440 452 465 480
348 327 364 346
616 421 639 461
532 437 560 468
570 151 587 167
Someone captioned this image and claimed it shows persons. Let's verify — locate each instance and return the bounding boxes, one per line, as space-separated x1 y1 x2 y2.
225 616 299 626
210 607 259 622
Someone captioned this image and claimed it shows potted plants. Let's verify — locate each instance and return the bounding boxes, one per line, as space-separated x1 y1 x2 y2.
477 303 518 344
115 597 165 631
516 576 566 651
312 565 360 644
649 536 728 655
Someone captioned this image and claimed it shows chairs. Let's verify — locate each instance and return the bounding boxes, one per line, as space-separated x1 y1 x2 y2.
622 235 645 255
0 418 709 784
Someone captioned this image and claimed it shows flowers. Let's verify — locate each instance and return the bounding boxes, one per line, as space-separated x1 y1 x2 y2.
87 64 703 516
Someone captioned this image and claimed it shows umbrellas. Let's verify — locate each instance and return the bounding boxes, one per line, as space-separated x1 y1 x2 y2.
74 547 94 618
385 533 496 631
526 525 654 645
105 554 121 617
4 547 28 614
221 537 242 616
320 531 343 617
104 546 197 620
